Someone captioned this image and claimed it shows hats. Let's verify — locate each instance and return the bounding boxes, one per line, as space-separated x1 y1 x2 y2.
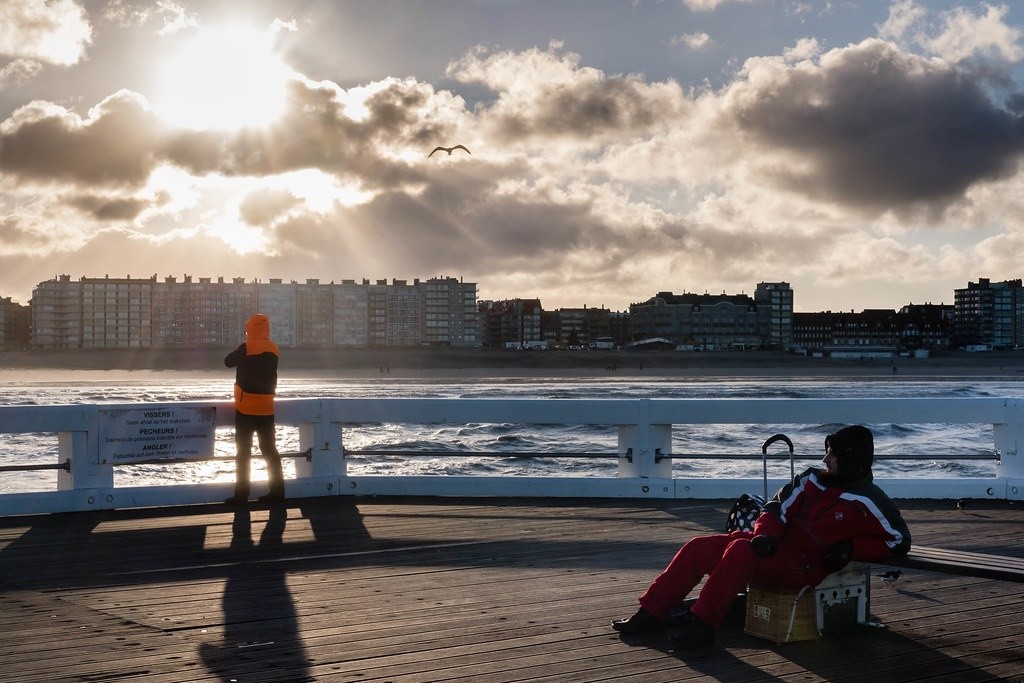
825 425 874 482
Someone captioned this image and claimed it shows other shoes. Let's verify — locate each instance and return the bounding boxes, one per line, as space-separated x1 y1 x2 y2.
610 606 668 632
258 493 285 505
669 616 717 644
225 494 249 506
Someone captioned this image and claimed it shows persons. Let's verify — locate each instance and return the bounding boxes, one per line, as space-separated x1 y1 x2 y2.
613 426 912 640
224 315 287 503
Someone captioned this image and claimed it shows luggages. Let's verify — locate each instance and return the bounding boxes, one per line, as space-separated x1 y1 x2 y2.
725 433 794 533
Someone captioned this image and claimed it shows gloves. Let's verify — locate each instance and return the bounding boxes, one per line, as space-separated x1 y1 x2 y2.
749 534 777 557
821 538 854 567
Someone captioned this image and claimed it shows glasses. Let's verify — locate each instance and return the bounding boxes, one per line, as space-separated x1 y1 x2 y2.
244 331 248 336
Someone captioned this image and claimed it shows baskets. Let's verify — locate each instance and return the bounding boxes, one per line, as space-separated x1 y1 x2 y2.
743 583 820 646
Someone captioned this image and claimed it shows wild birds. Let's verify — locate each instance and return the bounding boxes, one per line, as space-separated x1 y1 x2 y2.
428 144 471 158
